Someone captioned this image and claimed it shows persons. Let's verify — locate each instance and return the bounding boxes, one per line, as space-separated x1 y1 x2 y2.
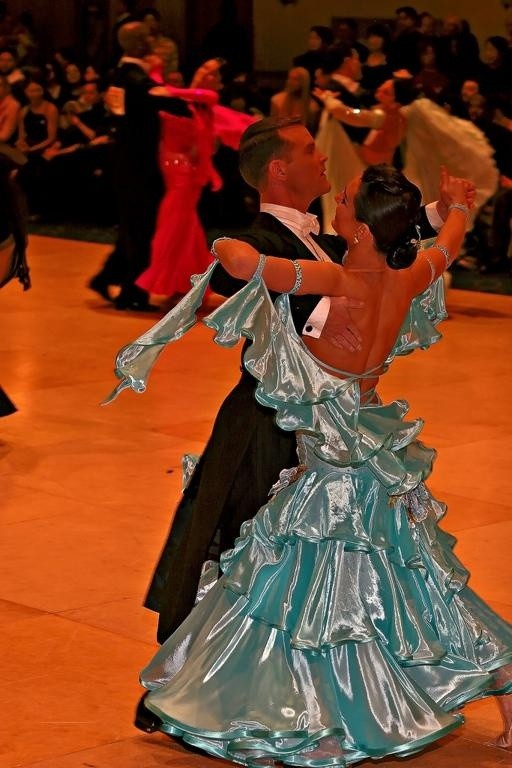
131 113 468 755
102 161 512 767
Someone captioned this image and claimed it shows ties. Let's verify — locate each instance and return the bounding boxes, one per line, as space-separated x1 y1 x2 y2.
296 215 324 237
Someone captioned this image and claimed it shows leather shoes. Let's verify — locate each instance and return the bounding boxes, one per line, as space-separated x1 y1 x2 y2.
134 691 204 755
